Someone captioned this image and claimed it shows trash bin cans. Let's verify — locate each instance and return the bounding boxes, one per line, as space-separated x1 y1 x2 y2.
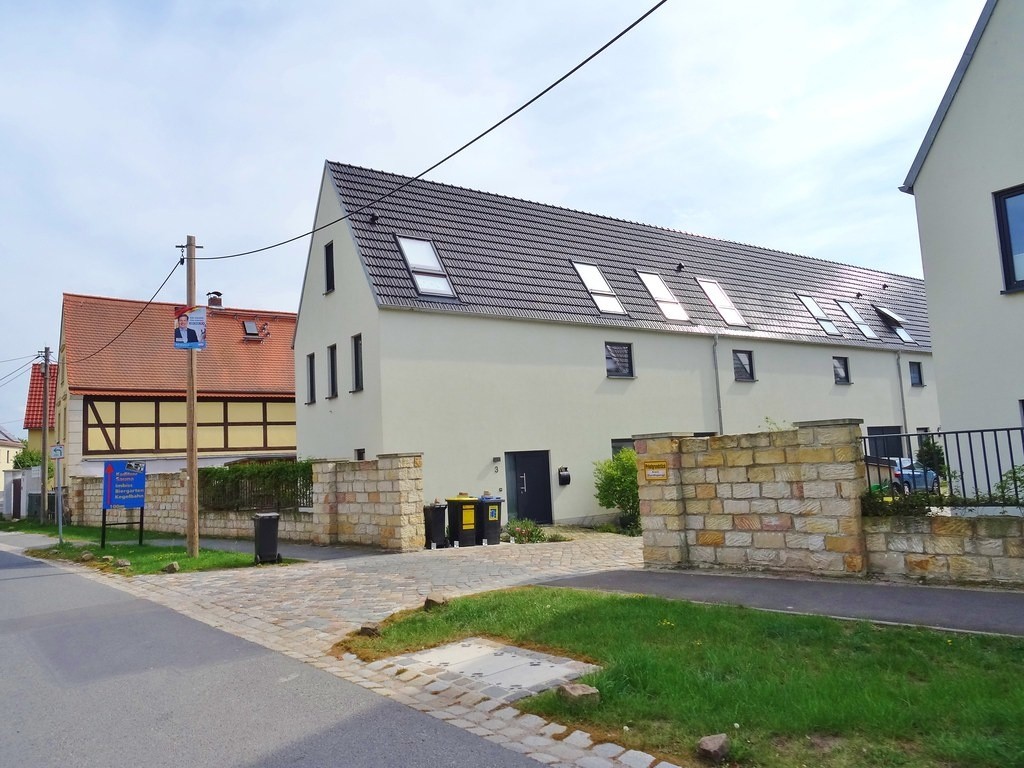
423 504 449 548
250 512 282 564
477 495 505 547
864 455 898 488
445 495 479 549
28 492 64 520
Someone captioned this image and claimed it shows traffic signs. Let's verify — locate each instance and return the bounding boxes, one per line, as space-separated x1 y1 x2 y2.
103 461 146 508
50 445 64 460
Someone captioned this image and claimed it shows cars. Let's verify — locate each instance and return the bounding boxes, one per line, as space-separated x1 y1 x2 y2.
881 456 939 497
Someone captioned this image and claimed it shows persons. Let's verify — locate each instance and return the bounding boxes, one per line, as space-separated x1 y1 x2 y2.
175 313 199 342
